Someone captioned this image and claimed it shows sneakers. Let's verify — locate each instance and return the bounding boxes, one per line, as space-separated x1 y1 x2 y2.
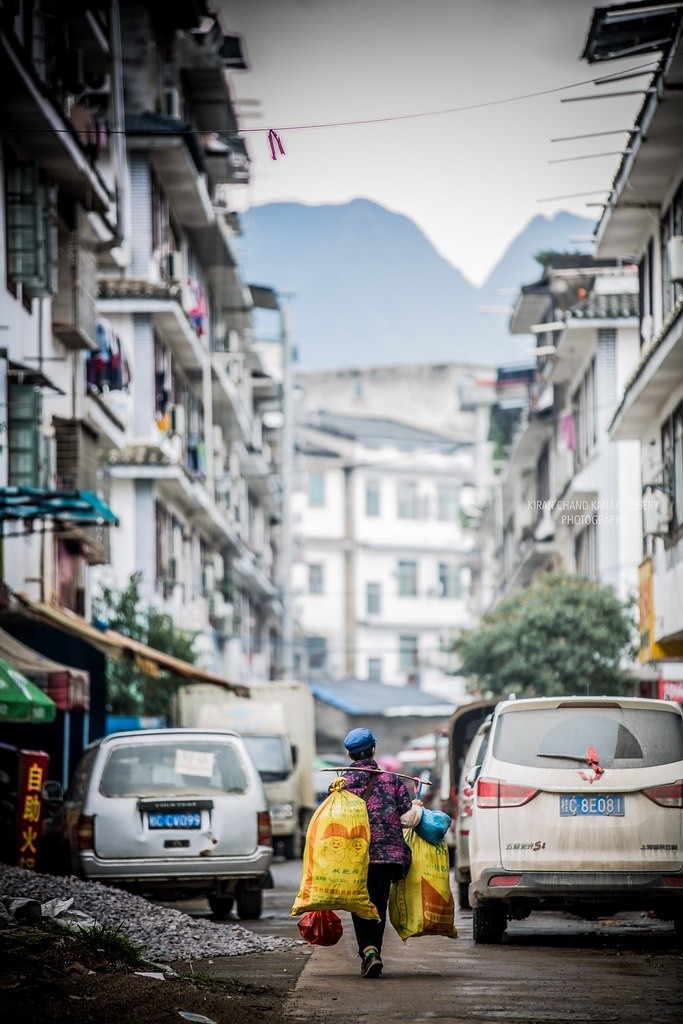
360 953 383 979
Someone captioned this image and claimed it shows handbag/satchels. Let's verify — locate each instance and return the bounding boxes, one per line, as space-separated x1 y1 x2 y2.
415 780 452 845
398 777 425 828
297 909 343 946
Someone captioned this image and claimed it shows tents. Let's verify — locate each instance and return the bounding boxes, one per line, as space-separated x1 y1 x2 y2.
0 626 89 791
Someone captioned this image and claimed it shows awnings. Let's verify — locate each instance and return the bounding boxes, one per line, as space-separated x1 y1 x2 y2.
0 486 121 538
13 590 251 698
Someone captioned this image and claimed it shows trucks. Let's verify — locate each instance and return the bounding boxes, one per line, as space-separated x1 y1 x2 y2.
174 680 320 859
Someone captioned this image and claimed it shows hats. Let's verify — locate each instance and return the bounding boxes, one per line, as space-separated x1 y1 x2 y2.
344 728 377 754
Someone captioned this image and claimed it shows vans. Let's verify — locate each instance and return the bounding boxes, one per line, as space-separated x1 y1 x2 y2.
40 727 276 920
462 691 683 945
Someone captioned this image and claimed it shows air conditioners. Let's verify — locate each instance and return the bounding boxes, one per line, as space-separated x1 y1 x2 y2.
642 483 671 535
667 236 683 281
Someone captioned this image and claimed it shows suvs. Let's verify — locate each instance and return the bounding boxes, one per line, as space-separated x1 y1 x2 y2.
314 696 494 910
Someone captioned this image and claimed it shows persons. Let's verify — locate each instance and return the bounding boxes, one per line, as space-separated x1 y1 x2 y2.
327 728 424 978
